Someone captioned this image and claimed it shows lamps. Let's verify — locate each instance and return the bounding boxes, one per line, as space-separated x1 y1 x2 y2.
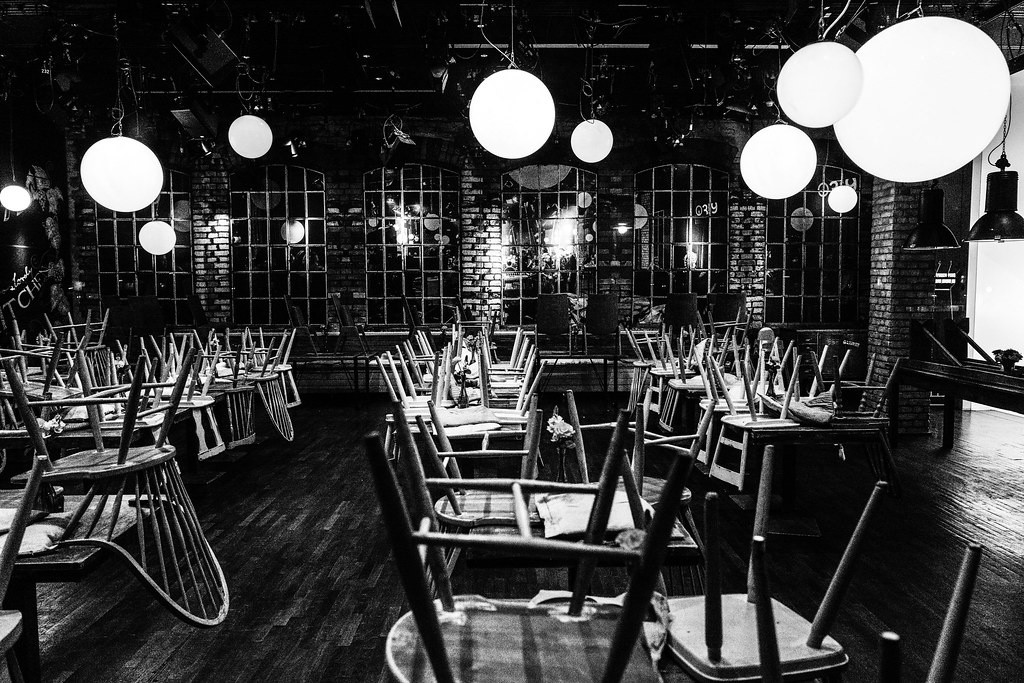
0 0 1024 257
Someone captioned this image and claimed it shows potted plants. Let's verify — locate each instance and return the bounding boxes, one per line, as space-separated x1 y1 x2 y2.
993 349 1023 372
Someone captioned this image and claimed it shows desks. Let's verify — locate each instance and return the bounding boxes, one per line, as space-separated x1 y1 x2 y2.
471 509 707 593
391 394 528 513
0 381 289 484
0 488 171 682
886 356 1024 450
745 419 910 525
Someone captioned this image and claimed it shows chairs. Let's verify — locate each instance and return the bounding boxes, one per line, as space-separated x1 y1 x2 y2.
0 301 986 683
